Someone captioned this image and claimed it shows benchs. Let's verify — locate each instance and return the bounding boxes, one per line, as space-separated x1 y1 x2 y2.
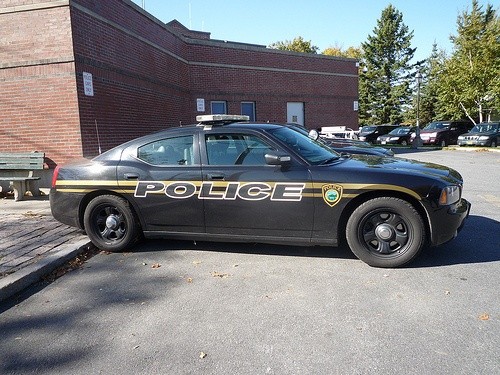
0 150 45 201
319 126 361 140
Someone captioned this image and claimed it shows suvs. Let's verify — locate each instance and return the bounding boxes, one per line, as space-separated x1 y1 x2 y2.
357 125 400 144
410 120 468 148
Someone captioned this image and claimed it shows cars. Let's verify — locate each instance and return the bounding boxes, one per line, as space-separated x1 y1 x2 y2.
457 122 500 147
48 114 471 269
281 121 394 157
376 126 421 146
